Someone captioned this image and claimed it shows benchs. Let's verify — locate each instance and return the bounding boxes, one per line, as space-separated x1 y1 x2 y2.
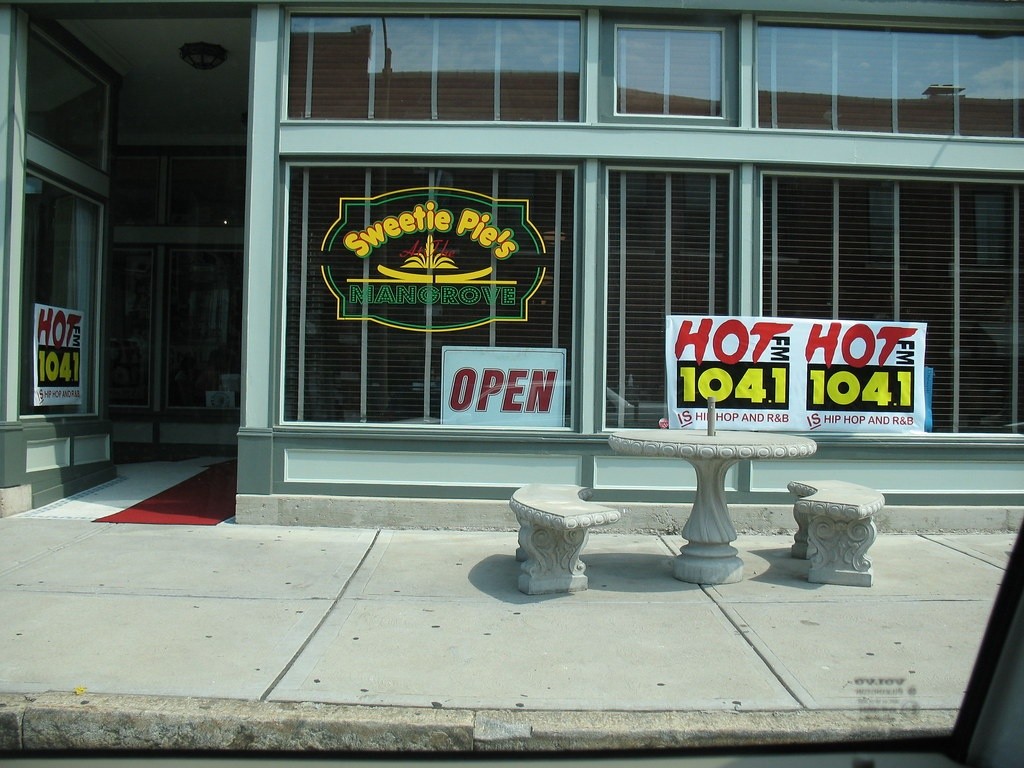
508 483 621 596
786 479 886 588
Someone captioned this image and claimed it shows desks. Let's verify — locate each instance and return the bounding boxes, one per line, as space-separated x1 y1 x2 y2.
608 429 818 585
